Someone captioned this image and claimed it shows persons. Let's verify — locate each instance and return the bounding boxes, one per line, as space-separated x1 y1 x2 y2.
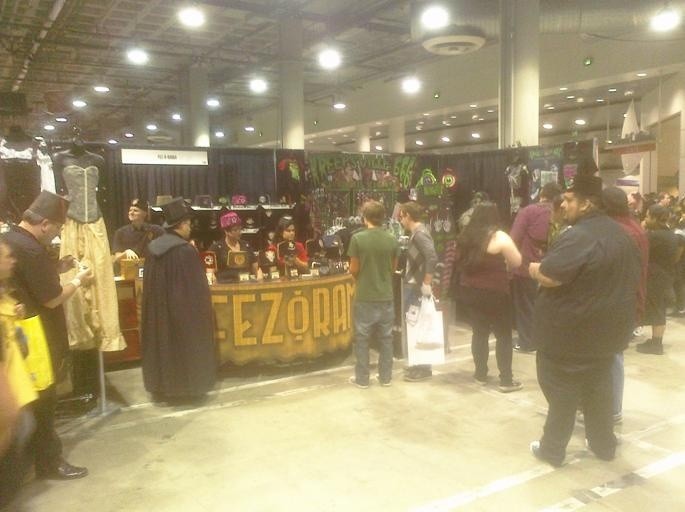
399 202 438 381
1 241 36 509
53 124 129 354
0 190 89 479
142 198 220 406
112 199 165 263
269 216 309 271
209 212 263 280
456 173 685 393
510 174 649 468
347 201 401 388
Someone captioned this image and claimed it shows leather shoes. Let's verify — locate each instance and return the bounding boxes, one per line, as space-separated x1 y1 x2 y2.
35 463 87 479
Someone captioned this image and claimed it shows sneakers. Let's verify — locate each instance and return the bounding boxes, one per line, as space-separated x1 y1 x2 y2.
532 408 622 467
510 311 685 355
349 365 523 392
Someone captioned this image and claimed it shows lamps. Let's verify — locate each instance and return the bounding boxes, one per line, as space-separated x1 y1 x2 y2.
423 25 485 56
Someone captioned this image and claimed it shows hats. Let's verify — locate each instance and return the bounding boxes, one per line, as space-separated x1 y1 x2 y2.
566 174 603 196
220 212 241 228
28 189 70 223
160 197 200 229
131 199 148 213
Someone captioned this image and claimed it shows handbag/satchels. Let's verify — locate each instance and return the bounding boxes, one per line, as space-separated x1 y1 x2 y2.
403 311 445 366
456 257 485 275
14 314 53 391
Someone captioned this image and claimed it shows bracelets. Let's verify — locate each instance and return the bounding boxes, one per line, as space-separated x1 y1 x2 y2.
69 278 84 289
